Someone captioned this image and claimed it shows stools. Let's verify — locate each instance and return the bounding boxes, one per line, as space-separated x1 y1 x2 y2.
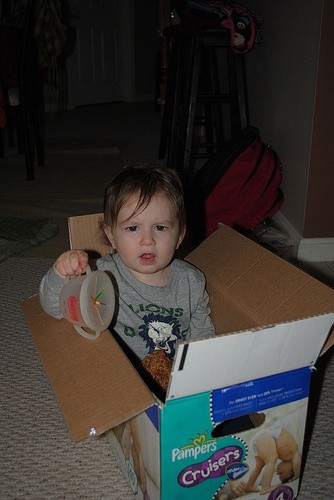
160 16 248 186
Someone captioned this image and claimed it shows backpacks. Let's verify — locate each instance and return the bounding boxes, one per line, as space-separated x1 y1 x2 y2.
189 126 286 244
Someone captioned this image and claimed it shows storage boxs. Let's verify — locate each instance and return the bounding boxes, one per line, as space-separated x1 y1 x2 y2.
20 214 334 500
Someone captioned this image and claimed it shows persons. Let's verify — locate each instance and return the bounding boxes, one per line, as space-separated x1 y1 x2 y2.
245 424 301 494
39 162 216 360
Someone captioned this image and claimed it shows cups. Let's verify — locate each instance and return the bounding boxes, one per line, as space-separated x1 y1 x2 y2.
58 264 115 340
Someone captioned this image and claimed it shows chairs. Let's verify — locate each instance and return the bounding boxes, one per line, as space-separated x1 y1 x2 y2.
0 0 44 181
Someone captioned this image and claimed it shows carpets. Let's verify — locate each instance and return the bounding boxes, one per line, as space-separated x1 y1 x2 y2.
0 256 334 500
